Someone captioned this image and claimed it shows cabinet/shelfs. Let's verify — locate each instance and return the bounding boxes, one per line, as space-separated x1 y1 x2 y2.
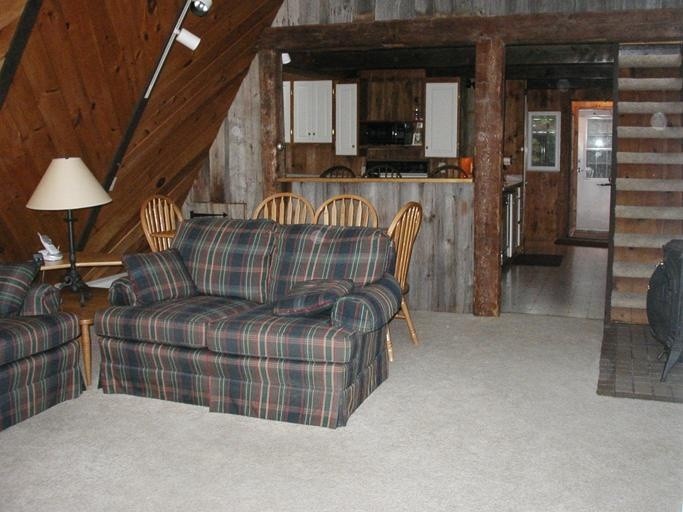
292 72 334 144
333 76 462 159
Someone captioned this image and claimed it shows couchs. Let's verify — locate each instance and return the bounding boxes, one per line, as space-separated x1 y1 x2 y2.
97 212 401 427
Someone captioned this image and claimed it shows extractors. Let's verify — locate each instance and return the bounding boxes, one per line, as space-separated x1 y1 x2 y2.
357 120 423 149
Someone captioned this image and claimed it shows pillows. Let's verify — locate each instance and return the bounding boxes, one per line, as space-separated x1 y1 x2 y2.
120 246 200 308
0 261 39 320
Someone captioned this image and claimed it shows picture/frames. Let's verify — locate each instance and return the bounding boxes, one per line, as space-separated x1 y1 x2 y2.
527 109 560 172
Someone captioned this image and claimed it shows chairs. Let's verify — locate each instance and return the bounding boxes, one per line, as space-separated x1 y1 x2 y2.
140 194 182 253
248 190 424 361
427 165 467 180
0 260 85 430
320 164 402 179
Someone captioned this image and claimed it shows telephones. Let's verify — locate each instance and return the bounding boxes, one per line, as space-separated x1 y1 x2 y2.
36 232 64 261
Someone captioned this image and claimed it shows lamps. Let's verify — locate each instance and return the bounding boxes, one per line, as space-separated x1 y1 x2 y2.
24 151 114 308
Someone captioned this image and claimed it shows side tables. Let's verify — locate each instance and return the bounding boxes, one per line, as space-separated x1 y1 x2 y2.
51 284 114 385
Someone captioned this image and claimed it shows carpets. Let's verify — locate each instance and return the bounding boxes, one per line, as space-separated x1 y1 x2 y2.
0 304 682 510
513 253 564 267
553 236 609 249
596 322 682 404
